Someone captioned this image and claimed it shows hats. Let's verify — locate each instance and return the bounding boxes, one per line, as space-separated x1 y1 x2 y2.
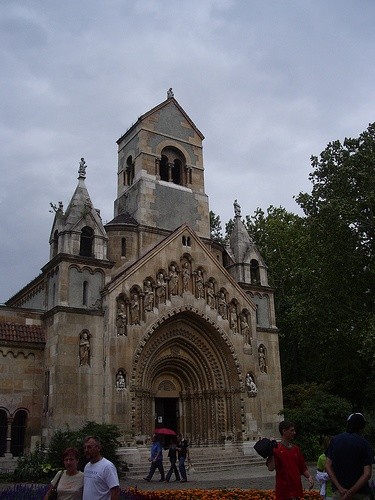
346 412 365 425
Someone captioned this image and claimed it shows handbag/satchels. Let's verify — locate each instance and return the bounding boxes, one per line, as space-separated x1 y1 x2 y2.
254 438 278 458
48 470 64 500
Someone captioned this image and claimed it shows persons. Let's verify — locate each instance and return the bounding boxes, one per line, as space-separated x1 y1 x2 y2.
246 373 259 392
43 447 84 500
165 436 181 482
116 256 251 354
175 433 191 482
83 435 122 500
266 420 314 500
325 412 373 500
79 332 90 366
315 448 330 500
143 433 165 482
116 371 125 388
259 346 266 373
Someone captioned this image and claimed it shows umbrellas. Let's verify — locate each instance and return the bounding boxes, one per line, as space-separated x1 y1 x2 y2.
153 427 177 435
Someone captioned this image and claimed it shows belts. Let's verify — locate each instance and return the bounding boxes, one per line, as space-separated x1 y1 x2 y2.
317 468 327 472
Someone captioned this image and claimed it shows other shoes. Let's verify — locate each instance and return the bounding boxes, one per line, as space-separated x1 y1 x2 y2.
163 480 169 483
174 479 187 482
159 479 165 482
143 477 151 482
